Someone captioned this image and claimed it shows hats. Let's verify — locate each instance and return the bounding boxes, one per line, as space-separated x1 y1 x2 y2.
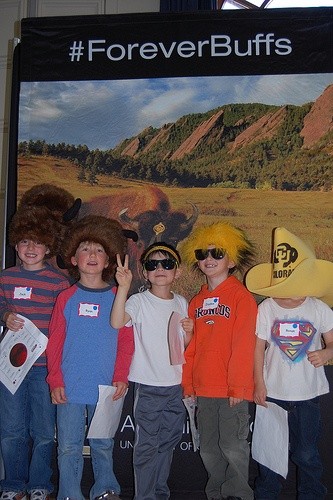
246 228 333 298
10 184 81 260
57 216 138 281
140 241 181 268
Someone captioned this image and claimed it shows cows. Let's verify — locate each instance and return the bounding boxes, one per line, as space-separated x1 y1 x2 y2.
78 185 200 296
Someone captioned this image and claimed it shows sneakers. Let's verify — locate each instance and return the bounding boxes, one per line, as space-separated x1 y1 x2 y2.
30 489 48 500
0 490 26 500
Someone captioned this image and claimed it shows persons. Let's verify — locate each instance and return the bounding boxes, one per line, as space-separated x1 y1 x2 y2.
245 227 333 500
0 206 71 500
46 214 135 500
176 220 254 500
110 241 194 500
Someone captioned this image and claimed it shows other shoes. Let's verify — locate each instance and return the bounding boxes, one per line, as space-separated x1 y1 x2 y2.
95 491 119 500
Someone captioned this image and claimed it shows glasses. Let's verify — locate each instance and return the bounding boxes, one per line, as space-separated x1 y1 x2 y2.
195 247 227 261
144 259 175 272
